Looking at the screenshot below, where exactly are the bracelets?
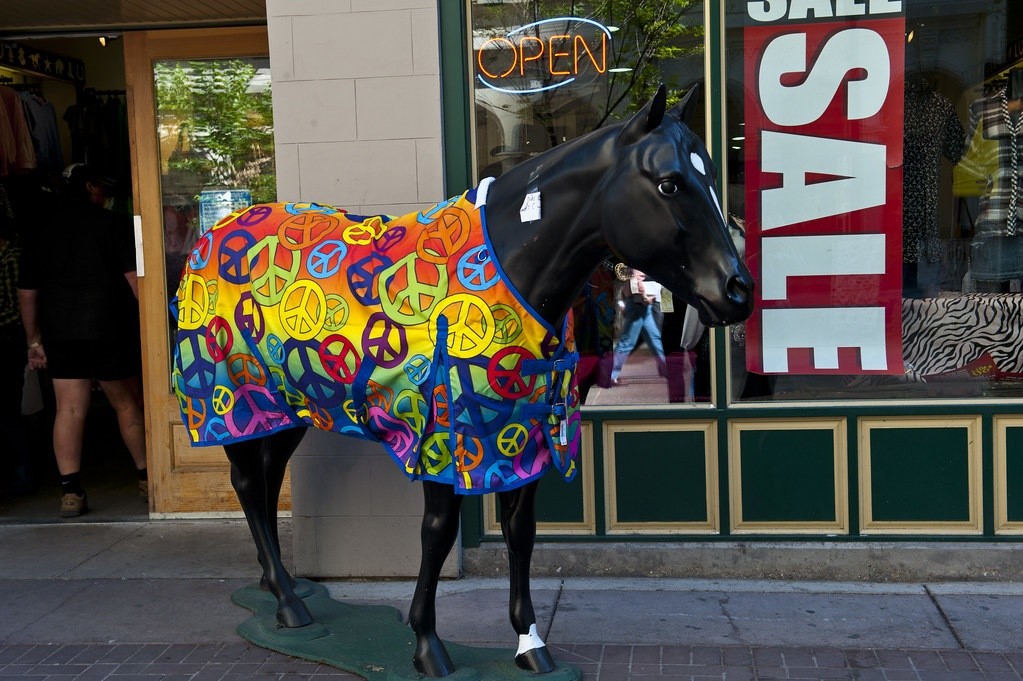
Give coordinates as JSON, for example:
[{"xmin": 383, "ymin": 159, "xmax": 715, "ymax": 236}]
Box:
[{"xmin": 28, "ymin": 340, "xmax": 42, "ymax": 348}]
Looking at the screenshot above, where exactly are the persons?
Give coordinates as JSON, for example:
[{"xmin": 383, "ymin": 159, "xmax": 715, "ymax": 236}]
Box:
[
  {"xmin": 966, "ymin": 79, "xmax": 1023, "ymax": 283},
  {"xmin": 611, "ymin": 269, "xmax": 667, "ymax": 386},
  {"xmin": 18, "ymin": 167, "xmax": 149, "ymax": 518},
  {"xmin": 902, "ymin": 73, "xmax": 967, "ymax": 263}
]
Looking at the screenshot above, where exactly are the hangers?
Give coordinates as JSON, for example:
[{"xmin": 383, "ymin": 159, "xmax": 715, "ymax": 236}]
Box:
[
  {"xmin": 0, "ymin": 74, "xmax": 47, "ymax": 104},
  {"xmin": 92, "ymin": 88, "xmax": 125, "ymax": 103}
]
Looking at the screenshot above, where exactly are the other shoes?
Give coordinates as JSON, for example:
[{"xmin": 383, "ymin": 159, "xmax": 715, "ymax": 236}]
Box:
[
  {"xmin": 60, "ymin": 494, "xmax": 88, "ymax": 516},
  {"xmin": 138, "ymin": 481, "xmax": 149, "ymax": 504},
  {"xmin": 611, "ymin": 377, "xmax": 626, "ymax": 385}
]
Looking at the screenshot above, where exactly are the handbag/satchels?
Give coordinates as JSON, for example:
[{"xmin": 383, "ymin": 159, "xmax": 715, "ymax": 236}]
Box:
[{"xmin": 633, "ymin": 281, "xmax": 662, "ymax": 303}]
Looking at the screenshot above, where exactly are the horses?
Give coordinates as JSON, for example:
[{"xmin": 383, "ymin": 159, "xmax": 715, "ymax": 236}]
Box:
[{"xmin": 171, "ymin": 80, "xmax": 755, "ymax": 679}]
